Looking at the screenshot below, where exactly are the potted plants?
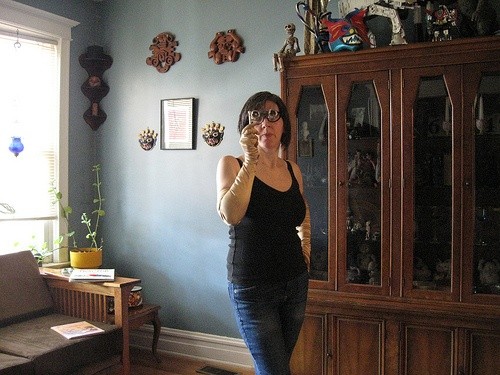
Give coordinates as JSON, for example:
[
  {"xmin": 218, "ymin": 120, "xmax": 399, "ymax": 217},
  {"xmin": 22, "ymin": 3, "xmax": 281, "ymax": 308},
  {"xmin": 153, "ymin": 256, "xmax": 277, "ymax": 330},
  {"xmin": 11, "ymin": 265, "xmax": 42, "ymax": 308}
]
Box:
[{"xmin": 14, "ymin": 164, "xmax": 105, "ymax": 269}]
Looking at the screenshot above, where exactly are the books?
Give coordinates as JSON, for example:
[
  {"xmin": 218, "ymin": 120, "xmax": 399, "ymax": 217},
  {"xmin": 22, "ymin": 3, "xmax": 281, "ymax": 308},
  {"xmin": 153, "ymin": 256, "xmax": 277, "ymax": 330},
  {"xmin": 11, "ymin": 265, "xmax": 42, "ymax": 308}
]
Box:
[
  {"xmin": 51, "ymin": 321, "xmax": 105, "ymax": 339},
  {"xmin": 69, "ymin": 269, "xmax": 115, "ymax": 282}
]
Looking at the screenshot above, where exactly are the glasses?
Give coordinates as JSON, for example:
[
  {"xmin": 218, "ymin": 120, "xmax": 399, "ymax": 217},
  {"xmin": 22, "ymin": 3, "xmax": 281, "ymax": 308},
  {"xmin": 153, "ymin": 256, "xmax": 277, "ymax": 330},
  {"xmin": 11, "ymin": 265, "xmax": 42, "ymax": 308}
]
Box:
[{"xmin": 250, "ymin": 111, "xmax": 282, "ymax": 125}]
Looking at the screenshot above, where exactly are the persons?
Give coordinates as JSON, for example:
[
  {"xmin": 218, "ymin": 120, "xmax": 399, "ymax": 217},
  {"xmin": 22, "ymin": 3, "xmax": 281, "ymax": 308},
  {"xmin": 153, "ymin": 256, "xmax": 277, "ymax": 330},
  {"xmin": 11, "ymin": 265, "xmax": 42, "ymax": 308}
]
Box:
[{"xmin": 217, "ymin": 90, "xmax": 311, "ymax": 374}]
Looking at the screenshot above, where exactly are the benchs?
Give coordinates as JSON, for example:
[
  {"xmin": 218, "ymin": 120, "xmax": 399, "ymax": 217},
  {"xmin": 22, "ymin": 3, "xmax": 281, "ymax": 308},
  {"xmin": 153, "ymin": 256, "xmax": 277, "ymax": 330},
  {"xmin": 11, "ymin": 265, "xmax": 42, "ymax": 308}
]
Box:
[{"xmin": 0, "ymin": 250, "xmax": 141, "ymax": 375}]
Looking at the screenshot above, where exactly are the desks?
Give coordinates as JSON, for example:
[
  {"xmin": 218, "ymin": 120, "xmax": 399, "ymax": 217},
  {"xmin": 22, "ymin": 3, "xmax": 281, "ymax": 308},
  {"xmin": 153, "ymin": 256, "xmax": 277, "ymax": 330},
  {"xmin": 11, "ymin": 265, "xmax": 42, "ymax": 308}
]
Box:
[{"xmin": 107, "ymin": 303, "xmax": 162, "ymax": 364}]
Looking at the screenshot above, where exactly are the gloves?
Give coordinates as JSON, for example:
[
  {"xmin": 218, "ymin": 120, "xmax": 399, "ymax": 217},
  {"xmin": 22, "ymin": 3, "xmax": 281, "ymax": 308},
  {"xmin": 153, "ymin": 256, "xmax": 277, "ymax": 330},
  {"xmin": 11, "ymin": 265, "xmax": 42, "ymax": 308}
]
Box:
[
  {"xmin": 218, "ymin": 121, "xmax": 260, "ymax": 226},
  {"xmin": 296, "ymin": 206, "xmax": 311, "ymax": 272}
]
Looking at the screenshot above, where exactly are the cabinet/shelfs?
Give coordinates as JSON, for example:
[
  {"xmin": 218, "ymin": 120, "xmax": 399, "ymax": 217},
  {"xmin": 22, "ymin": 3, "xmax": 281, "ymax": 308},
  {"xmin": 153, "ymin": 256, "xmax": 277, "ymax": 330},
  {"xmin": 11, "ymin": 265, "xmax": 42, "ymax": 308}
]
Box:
[
  {"xmin": 280, "ymin": 37, "xmax": 500, "ymax": 375},
  {"xmin": 79, "ymin": 44, "xmax": 112, "ymax": 131}
]
